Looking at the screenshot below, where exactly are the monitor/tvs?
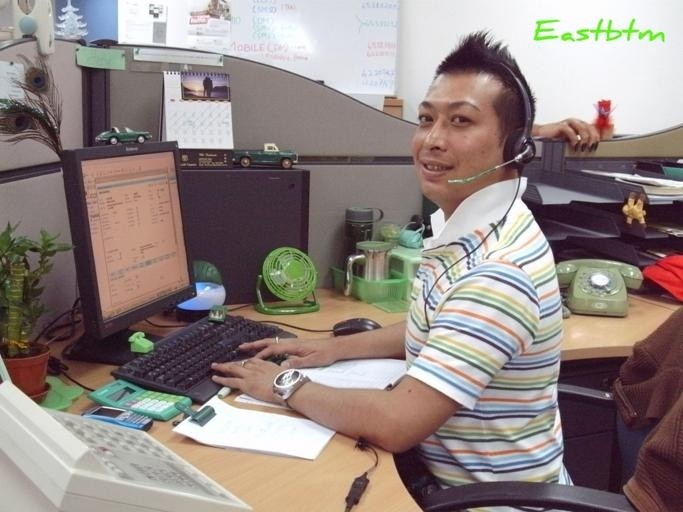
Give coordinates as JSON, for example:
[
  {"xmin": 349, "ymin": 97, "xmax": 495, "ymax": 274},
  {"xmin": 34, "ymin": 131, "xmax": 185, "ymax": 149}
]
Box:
[{"xmin": 58, "ymin": 140, "xmax": 199, "ymax": 366}]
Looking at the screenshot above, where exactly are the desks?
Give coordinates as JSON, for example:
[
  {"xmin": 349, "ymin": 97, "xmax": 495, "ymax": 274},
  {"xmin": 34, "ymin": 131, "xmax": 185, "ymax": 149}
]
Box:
[{"xmin": 32, "ymin": 270, "xmax": 683, "ymax": 512}]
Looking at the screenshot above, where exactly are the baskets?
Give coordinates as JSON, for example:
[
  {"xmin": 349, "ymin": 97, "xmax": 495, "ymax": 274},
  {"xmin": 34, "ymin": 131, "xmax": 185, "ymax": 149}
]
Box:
[{"xmin": 329, "ymin": 266, "xmax": 409, "ymax": 303}]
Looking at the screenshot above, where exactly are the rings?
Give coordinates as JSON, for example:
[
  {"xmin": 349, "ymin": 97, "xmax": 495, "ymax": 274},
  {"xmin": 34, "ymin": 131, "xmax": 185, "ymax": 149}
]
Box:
[
  {"xmin": 276, "ymin": 336, "xmax": 279, "ymax": 344},
  {"xmin": 242, "ymin": 360, "xmax": 250, "ymax": 366}
]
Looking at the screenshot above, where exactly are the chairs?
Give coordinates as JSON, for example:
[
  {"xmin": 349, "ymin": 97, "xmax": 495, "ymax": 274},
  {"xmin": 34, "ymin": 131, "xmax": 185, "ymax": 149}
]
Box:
[{"xmin": 418, "ymin": 304, "xmax": 683, "ymax": 511}]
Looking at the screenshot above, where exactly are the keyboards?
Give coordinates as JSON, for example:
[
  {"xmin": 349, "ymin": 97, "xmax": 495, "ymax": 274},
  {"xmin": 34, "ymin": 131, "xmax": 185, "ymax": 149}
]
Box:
[{"xmin": 109, "ymin": 312, "xmax": 297, "ymax": 405}]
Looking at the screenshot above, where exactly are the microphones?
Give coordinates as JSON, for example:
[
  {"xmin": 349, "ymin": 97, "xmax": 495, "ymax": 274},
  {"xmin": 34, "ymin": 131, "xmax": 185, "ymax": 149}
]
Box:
[{"xmin": 447, "ymin": 159, "xmax": 513, "ymax": 186}]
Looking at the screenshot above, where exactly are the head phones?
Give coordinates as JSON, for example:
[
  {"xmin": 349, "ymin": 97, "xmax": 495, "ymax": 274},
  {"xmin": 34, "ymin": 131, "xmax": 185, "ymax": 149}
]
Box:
[{"xmin": 500, "ymin": 61, "xmax": 539, "ymax": 173}]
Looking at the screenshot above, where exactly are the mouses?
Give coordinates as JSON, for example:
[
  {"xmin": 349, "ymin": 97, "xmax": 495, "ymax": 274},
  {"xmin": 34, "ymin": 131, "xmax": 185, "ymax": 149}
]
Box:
[{"xmin": 333, "ymin": 315, "xmax": 380, "ymax": 336}]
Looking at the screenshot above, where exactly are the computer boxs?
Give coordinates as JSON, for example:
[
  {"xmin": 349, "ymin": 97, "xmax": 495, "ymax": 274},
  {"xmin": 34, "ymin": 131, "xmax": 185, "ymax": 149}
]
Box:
[{"xmin": 173, "ymin": 166, "xmax": 310, "ymax": 302}]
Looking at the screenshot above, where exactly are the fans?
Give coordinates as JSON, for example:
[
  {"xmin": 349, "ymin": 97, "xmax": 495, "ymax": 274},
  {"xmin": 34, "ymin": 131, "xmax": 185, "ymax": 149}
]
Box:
[{"xmin": 255, "ymin": 248, "xmax": 321, "ymax": 313}]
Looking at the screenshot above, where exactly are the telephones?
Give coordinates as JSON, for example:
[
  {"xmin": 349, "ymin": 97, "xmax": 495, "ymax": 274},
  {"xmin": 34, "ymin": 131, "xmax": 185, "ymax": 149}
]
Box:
[
  {"xmin": 556, "ymin": 257, "xmax": 643, "ymax": 318},
  {"xmin": 128, "ymin": 331, "xmax": 154, "ymax": 353}
]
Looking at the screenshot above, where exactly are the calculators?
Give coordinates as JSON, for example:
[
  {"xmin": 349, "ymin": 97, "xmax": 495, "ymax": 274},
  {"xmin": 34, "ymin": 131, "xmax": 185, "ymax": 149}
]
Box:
[{"xmin": 89, "ymin": 380, "xmax": 191, "ymax": 420}]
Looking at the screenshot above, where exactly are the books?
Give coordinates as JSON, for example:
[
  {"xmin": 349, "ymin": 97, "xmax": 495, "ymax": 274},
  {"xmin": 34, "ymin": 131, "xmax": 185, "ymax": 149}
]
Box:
[{"xmin": 585, "ymin": 170, "xmax": 683, "ymax": 199}]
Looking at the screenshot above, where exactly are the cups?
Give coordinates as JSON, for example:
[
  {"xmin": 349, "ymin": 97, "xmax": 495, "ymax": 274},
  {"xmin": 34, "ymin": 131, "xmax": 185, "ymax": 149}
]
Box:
[{"xmin": 343, "ymin": 240, "xmax": 394, "ymax": 297}]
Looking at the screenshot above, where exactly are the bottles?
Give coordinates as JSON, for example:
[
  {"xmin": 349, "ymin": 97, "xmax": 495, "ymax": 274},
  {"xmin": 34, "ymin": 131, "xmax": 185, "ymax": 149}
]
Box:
[{"xmin": 342, "ymin": 206, "xmax": 384, "ymax": 276}]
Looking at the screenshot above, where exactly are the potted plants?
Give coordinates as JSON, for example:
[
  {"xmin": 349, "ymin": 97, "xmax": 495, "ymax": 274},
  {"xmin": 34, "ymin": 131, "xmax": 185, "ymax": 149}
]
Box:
[{"xmin": 0, "ymin": 221, "xmax": 76, "ymax": 404}]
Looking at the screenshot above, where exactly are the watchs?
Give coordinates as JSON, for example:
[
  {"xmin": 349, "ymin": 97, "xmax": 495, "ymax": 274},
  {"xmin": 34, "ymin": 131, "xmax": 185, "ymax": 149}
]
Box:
[{"xmin": 272, "ymin": 369, "xmax": 312, "ymax": 409}]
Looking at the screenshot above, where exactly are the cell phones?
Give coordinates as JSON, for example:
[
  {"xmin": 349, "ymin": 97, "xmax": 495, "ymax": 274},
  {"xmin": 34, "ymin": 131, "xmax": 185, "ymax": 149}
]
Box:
[{"xmin": 81, "ymin": 404, "xmax": 153, "ymax": 430}]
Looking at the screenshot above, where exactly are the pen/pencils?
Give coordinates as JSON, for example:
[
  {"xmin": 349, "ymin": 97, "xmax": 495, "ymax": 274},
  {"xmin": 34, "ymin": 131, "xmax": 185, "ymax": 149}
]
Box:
[{"xmin": 218, "ymin": 386, "xmax": 232, "ymax": 399}]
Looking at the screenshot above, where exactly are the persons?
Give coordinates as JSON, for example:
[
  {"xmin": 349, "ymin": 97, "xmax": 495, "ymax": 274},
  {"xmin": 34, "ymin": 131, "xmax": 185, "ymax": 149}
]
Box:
[
  {"xmin": 531, "ymin": 119, "xmax": 600, "ymax": 153},
  {"xmin": 211, "ymin": 23, "xmax": 575, "ymax": 512},
  {"xmin": 94, "ymin": 127, "xmax": 152, "ymax": 145},
  {"xmin": 232, "ymin": 143, "xmax": 296, "ymax": 165},
  {"xmin": 203, "ymin": 76, "xmax": 213, "ymax": 98}
]
